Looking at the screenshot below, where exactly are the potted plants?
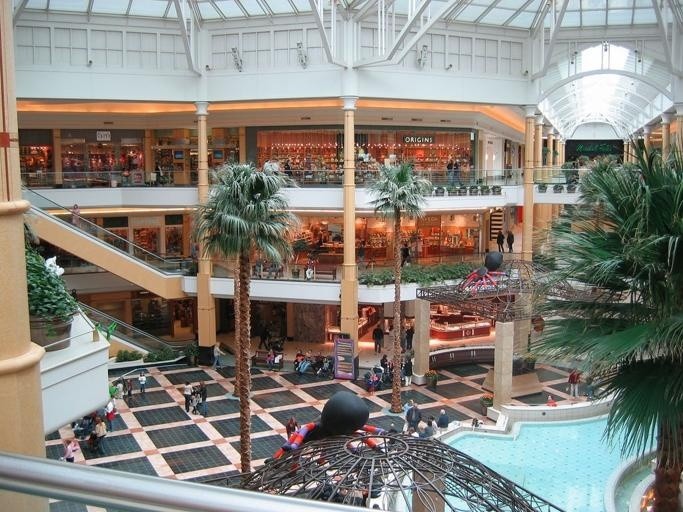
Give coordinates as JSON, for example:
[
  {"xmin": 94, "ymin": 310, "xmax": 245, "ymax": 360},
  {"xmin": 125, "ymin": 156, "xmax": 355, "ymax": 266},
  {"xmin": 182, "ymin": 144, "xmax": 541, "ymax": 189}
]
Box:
[
  {"xmin": 291, "ymin": 239, "xmax": 308, "ymax": 278},
  {"xmin": 538, "ymin": 183, "xmax": 577, "ymax": 193},
  {"xmin": 436, "ymin": 185, "xmax": 501, "ymax": 196},
  {"xmin": 26, "ymin": 244, "xmax": 79, "ymax": 352},
  {"xmin": 186, "ymin": 341, "xmax": 199, "ymax": 363}
]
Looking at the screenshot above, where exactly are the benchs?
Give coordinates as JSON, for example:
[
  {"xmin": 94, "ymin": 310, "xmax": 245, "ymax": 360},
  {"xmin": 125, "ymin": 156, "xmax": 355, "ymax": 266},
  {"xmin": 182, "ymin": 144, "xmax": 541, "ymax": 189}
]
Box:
[
  {"xmin": 293, "ymin": 356, "xmax": 335, "ymax": 379},
  {"xmin": 302, "ymin": 263, "xmax": 337, "ymax": 280},
  {"xmin": 252, "ymin": 261, "xmax": 284, "ymax": 279},
  {"xmin": 251, "ymin": 351, "xmax": 284, "ymax": 370}
]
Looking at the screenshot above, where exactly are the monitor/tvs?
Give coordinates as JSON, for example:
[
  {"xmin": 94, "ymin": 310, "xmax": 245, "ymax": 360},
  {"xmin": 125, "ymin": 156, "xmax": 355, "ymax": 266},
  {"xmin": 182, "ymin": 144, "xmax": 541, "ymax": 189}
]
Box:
[
  {"xmin": 174, "ymin": 151, "xmax": 184, "ymax": 159},
  {"xmin": 213, "ymin": 150, "xmax": 223, "ymax": 159}
]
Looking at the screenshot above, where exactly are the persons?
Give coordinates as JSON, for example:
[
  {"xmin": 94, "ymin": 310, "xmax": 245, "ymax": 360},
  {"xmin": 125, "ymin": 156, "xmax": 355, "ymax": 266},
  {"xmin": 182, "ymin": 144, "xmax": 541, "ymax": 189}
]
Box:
[
  {"xmin": 497, "ymin": 230, "xmax": 515, "ymax": 253},
  {"xmin": 287, "ymin": 418, "xmax": 301, "ymax": 439},
  {"xmin": 72, "ymin": 204, "xmax": 81, "ymax": 227},
  {"xmin": 547, "ymin": 395, "xmax": 556, "ymax": 406},
  {"xmin": 184, "ymin": 380, "xmax": 208, "ymax": 416},
  {"xmin": 262, "ymin": 157, "xmax": 292, "ymax": 178},
  {"xmin": 446, "ymin": 160, "xmax": 464, "ymax": 187},
  {"xmin": 568, "ymin": 369, "xmax": 593, "ymax": 398},
  {"xmin": 258, "ymin": 321, "xmax": 334, "ymax": 377},
  {"xmin": 358, "ymin": 244, "xmax": 364, "ymax": 265},
  {"xmin": 365, "ymin": 322, "xmax": 416, "ymax": 391},
  {"xmin": 155, "ymin": 162, "xmax": 164, "ymax": 185},
  {"xmin": 211, "ymin": 342, "xmax": 227, "ymax": 370},
  {"xmin": 401, "ymin": 244, "xmax": 412, "ymax": 267},
  {"xmin": 70, "ymin": 289, "xmax": 78, "ymax": 302},
  {"xmin": 390, "ymin": 398, "xmax": 448, "ymax": 438},
  {"xmin": 63, "ymin": 369, "xmax": 148, "ymax": 462}
]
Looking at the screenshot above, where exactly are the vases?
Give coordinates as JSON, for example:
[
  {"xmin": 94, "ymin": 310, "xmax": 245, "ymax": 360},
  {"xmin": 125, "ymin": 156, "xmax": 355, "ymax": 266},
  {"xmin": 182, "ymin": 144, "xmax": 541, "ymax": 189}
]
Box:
[
  {"xmin": 527, "ymin": 361, "xmax": 534, "ymax": 369},
  {"xmin": 426, "ymin": 376, "xmax": 437, "ymax": 388},
  {"xmin": 481, "ymin": 406, "xmax": 487, "ymax": 416}
]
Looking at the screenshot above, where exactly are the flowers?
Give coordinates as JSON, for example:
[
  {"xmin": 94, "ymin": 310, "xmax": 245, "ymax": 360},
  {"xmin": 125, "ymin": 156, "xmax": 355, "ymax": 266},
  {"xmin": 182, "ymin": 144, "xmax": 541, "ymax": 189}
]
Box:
[
  {"xmin": 425, "ymin": 370, "xmax": 437, "ymax": 377},
  {"xmin": 479, "ymin": 394, "xmax": 494, "ymax": 407},
  {"xmin": 525, "ymin": 353, "xmax": 537, "ymax": 362}
]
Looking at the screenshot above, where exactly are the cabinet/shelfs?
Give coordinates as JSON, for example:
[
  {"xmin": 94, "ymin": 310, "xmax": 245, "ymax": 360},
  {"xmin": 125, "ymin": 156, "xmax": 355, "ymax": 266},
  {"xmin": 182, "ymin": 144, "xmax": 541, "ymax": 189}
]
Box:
[{"xmin": 258, "ymin": 146, "xmax": 469, "ymax": 178}]
